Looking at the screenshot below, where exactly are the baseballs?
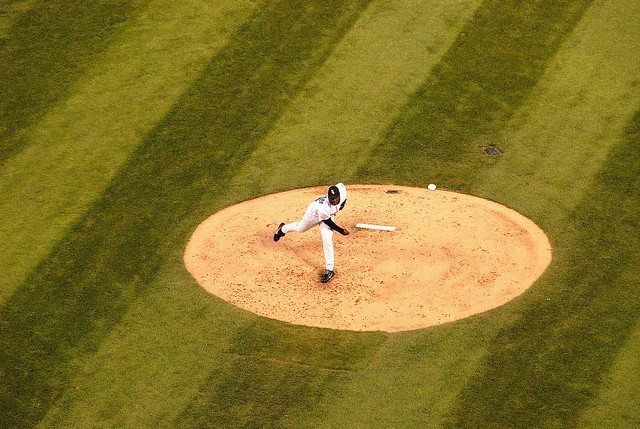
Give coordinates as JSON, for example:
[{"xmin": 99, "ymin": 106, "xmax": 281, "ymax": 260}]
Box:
[{"xmin": 428, "ymin": 184, "xmax": 436, "ymax": 190}]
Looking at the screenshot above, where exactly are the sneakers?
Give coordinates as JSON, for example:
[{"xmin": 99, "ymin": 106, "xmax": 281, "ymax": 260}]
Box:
[
  {"xmin": 321, "ymin": 269, "xmax": 333, "ymax": 282},
  {"xmin": 274, "ymin": 223, "xmax": 285, "ymax": 241}
]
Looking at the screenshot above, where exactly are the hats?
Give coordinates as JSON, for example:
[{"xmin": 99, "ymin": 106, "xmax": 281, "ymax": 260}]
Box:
[{"xmin": 328, "ymin": 186, "xmax": 339, "ymax": 198}]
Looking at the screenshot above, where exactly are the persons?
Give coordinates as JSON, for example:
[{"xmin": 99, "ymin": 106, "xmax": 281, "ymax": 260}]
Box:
[{"xmin": 273, "ymin": 183, "xmax": 349, "ymax": 283}]
